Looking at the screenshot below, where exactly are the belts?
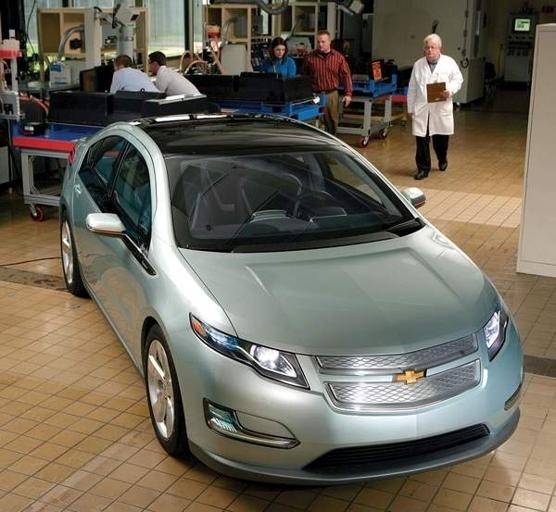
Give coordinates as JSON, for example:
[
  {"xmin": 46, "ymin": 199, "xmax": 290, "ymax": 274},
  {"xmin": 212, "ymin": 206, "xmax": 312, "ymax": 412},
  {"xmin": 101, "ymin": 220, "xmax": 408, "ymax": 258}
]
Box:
[{"xmin": 313, "ymin": 88, "xmax": 337, "ymax": 94}]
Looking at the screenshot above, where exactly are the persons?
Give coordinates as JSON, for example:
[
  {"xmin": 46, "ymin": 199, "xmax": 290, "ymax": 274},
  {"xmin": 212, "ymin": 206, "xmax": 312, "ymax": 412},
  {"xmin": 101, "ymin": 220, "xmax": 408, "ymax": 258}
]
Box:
[
  {"xmin": 262, "ymin": 38, "xmax": 296, "ymax": 118},
  {"xmin": 110, "ymin": 54, "xmax": 157, "ymax": 94},
  {"xmin": 300, "ymin": 30, "xmax": 354, "ymax": 136},
  {"xmin": 148, "ymin": 51, "xmax": 200, "ymax": 96},
  {"xmin": 406, "ymin": 32, "xmax": 464, "ymax": 180}
]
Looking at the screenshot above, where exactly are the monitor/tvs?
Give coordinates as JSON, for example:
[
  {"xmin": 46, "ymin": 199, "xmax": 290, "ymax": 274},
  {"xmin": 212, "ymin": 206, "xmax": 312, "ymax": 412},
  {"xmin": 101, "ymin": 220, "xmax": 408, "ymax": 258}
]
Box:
[
  {"xmin": 80, "ymin": 69, "xmax": 98, "ymax": 92},
  {"xmin": 508, "ymin": 13, "xmax": 536, "ymax": 43}
]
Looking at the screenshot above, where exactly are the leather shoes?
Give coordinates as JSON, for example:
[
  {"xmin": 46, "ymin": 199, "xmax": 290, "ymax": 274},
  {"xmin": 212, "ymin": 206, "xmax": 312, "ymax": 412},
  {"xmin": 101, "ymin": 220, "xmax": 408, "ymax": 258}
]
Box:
[
  {"xmin": 414, "ymin": 168, "xmax": 430, "ymax": 180},
  {"xmin": 437, "ymin": 157, "xmax": 449, "ymax": 171}
]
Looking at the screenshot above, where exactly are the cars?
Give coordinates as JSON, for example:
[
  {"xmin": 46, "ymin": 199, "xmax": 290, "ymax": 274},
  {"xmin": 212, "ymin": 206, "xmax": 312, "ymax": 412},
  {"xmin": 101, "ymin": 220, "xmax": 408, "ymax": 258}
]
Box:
[{"xmin": 57, "ymin": 111, "xmax": 526, "ymax": 483}]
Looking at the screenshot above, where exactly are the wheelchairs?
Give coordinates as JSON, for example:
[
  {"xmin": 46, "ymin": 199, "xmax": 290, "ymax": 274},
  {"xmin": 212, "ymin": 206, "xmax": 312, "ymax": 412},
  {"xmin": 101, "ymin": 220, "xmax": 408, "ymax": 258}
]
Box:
[{"xmin": 481, "ymin": 57, "xmax": 498, "ymax": 101}]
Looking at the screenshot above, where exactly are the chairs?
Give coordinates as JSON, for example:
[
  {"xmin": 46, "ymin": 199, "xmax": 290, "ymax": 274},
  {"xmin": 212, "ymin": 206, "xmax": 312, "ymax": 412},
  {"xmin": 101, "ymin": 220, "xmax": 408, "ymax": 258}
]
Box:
[{"xmin": 179, "ymin": 156, "xmax": 304, "ymax": 225}]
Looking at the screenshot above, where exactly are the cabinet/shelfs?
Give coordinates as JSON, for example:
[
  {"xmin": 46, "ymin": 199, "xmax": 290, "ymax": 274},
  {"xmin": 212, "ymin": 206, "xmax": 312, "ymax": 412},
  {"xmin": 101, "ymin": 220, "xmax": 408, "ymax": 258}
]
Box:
[
  {"xmin": 271, "ymin": 1, "xmax": 335, "ymax": 51},
  {"xmin": 35, "ymin": 6, "xmax": 149, "ymax": 80},
  {"xmin": 199, "ymin": 2, "xmax": 278, "ymax": 72}
]
